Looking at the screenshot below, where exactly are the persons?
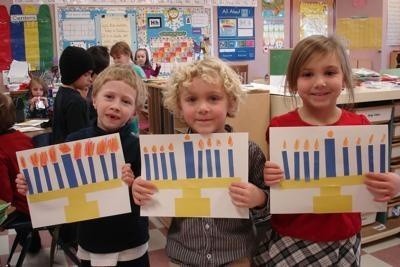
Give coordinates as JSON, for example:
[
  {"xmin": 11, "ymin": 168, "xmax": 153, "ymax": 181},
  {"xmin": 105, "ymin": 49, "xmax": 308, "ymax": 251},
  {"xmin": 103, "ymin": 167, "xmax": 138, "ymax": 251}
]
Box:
[
  {"xmin": 23, "ymin": 77, "xmax": 55, "ymax": 119},
  {"xmin": 109, "ymin": 41, "xmax": 146, "ymax": 135},
  {"xmin": 51, "ymin": 45, "xmax": 94, "ymax": 141},
  {"xmin": 16, "ymin": 63, "xmax": 151, "ymax": 266},
  {"xmin": 134, "ymin": 47, "xmax": 161, "ymax": 131},
  {"xmin": 253, "ymin": 34, "xmax": 400, "ymax": 266},
  {"xmin": 0, "ymin": 91, "xmax": 42, "ymax": 255},
  {"xmin": 86, "ymin": 45, "xmax": 110, "ymax": 100},
  {"xmin": 132, "ymin": 54, "xmax": 270, "ymax": 266}
]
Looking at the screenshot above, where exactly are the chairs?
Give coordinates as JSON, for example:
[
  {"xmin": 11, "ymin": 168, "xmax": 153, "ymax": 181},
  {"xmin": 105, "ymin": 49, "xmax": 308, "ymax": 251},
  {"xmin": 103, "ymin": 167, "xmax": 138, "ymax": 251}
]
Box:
[{"xmin": 0, "ymin": 129, "xmax": 84, "ymax": 267}]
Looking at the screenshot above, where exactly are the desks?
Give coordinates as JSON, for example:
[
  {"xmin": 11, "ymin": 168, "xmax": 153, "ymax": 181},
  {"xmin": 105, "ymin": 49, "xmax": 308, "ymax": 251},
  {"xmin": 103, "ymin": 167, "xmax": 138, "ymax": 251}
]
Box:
[{"xmin": 12, "ymin": 119, "xmax": 54, "ymax": 140}]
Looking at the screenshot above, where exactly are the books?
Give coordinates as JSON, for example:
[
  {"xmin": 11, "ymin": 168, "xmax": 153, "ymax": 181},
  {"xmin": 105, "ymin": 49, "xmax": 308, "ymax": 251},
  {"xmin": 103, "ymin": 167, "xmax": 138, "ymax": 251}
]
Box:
[{"xmin": 15, "ymin": 118, "xmax": 50, "ymax": 126}]
